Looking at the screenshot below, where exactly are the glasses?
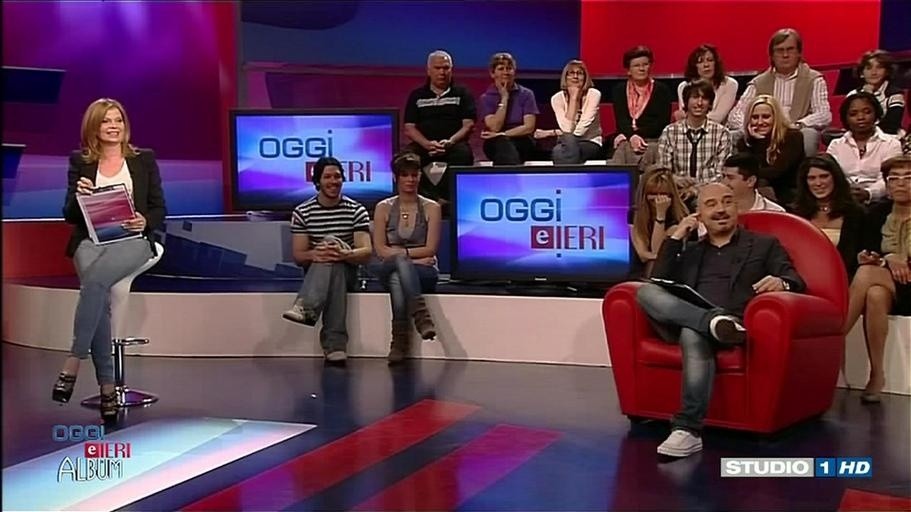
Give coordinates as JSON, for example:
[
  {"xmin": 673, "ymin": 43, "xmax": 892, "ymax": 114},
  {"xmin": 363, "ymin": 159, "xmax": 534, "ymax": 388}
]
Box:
[{"xmin": 886, "ymin": 175, "xmax": 911, "ymax": 183}]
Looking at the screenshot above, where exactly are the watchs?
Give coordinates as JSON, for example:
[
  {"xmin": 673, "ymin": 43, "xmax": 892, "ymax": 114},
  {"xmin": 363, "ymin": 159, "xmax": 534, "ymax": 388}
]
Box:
[
  {"xmin": 781, "ymin": 279, "xmax": 791, "ymax": 290},
  {"xmin": 655, "ymin": 219, "xmax": 666, "ymax": 225}
]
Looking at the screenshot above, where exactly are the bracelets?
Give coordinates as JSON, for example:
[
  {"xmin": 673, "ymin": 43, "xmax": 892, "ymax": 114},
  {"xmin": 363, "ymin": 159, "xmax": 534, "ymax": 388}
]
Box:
[
  {"xmin": 554, "ymin": 129, "xmax": 558, "ymax": 136},
  {"xmin": 498, "ymin": 104, "xmax": 507, "ymax": 107}
]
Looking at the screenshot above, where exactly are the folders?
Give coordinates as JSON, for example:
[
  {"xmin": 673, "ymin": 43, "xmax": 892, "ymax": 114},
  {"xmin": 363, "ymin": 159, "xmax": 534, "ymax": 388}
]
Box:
[{"xmin": 75, "ymin": 182, "xmax": 143, "ymax": 246}]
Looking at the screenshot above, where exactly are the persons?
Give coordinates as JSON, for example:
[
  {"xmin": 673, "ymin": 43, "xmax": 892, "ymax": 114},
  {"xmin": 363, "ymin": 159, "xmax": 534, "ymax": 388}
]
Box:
[
  {"xmin": 480, "ymin": 53, "xmax": 537, "ymax": 165},
  {"xmin": 825, "ymin": 92, "xmax": 903, "ymax": 205},
  {"xmin": 658, "ymin": 80, "xmax": 731, "ymax": 201},
  {"xmin": 841, "ymin": 155, "xmax": 911, "ymax": 405},
  {"xmin": 51, "ymin": 98, "xmax": 167, "ymax": 422},
  {"xmin": 607, "ymin": 45, "xmax": 672, "ymax": 165},
  {"xmin": 533, "ymin": 60, "xmax": 603, "ymax": 167},
  {"xmin": 733, "ymin": 94, "xmax": 806, "ymax": 201},
  {"xmin": 393, "ymin": 49, "xmax": 477, "ymax": 218},
  {"xmin": 280, "ymin": 156, "xmax": 373, "ymax": 363},
  {"xmin": 726, "ymin": 28, "xmax": 832, "ymax": 129},
  {"xmin": 628, "ymin": 169, "xmax": 699, "ymax": 267},
  {"xmin": 786, "ymin": 153, "xmax": 883, "ymax": 283},
  {"xmin": 637, "ymin": 182, "xmax": 807, "ymax": 457},
  {"xmin": 844, "ymin": 49, "xmax": 905, "ymax": 135},
  {"xmin": 374, "ymin": 150, "xmax": 441, "ymax": 363},
  {"xmin": 674, "ymin": 46, "xmax": 740, "ymax": 126},
  {"xmin": 718, "ymin": 157, "xmax": 783, "ymax": 211}
]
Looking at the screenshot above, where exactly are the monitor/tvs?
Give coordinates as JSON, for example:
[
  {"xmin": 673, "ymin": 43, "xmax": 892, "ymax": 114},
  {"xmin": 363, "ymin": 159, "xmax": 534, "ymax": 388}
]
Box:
[
  {"xmin": 446, "ymin": 164, "xmax": 637, "ymax": 293},
  {"xmin": 226, "ymin": 107, "xmax": 398, "ymax": 213}
]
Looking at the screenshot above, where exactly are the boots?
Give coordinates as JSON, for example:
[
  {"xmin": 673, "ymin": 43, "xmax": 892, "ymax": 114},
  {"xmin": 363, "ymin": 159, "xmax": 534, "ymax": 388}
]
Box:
[
  {"xmin": 409, "ymin": 295, "xmax": 436, "ymax": 341},
  {"xmin": 387, "ymin": 321, "xmax": 410, "ymax": 362}
]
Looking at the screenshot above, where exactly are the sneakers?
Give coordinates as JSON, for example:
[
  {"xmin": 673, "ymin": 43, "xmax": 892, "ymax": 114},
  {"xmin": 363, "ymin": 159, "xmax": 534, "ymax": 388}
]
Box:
[
  {"xmin": 657, "ymin": 430, "xmax": 702, "ymax": 457},
  {"xmin": 710, "ymin": 316, "xmax": 745, "ymax": 345},
  {"xmin": 282, "ymin": 299, "xmax": 315, "ymax": 327},
  {"xmin": 326, "ymin": 351, "xmax": 347, "ymax": 362}
]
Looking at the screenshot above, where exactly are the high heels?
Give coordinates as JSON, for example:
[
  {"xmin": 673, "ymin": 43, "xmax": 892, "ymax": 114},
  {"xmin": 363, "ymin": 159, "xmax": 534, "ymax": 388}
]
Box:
[
  {"xmin": 861, "ymin": 376, "xmax": 886, "ymax": 403},
  {"xmin": 101, "ymin": 387, "xmax": 120, "ymax": 421},
  {"xmin": 51, "ymin": 372, "xmax": 78, "ymax": 404}
]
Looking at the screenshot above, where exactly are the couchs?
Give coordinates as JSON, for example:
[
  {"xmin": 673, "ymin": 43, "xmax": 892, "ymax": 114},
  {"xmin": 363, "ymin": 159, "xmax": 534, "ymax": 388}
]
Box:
[{"xmin": 601, "ymin": 209, "xmax": 849, "ymax": 438}]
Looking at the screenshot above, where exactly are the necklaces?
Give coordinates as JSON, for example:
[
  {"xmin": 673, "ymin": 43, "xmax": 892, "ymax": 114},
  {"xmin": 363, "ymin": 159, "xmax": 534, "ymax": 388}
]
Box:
[
  {"xmin": 399, "ymin": 202, "xmax": 417, "ymax": 220},
  {"xmin": 817, "ymin": 206, "xmax": 830, "ymax": 212}
]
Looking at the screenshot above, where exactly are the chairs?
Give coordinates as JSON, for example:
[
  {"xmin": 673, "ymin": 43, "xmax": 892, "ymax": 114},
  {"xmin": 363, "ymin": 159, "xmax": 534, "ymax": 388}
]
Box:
[{"xmin": 79, "ymin": 241, "xmax": 163, "ymax": 407}]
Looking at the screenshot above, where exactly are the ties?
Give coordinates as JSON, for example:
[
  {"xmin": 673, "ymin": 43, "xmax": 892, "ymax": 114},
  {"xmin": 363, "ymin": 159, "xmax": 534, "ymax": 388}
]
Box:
[{"xmin": 687, "ymin": 130, "xmax": 705, "ymax": 178}]
